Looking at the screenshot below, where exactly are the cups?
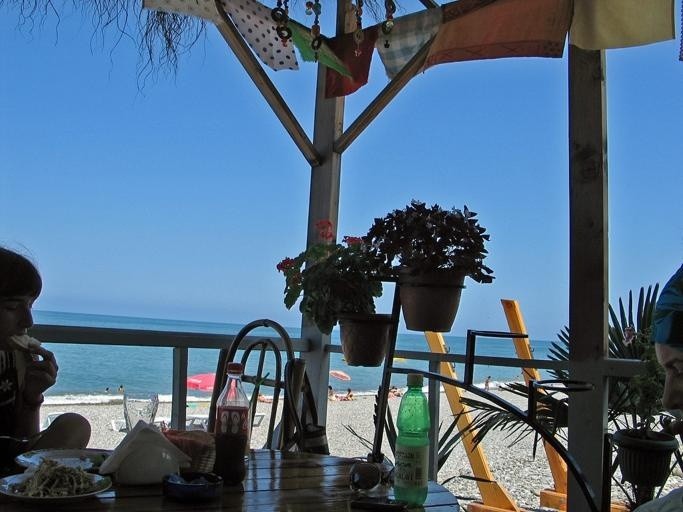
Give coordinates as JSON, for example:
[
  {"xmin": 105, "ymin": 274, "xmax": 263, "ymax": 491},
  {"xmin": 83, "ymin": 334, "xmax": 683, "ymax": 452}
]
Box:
[{"xmin": 123, "ymin": 392, "xmax": 158, "ymax": 430}]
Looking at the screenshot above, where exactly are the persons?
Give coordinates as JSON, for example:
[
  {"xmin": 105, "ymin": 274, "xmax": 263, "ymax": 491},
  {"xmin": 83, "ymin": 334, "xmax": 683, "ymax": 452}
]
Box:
[
  {"xmin": 631, "ymin": 264, "xmax": 683, "ymax": 512},
  {"xmin": 327, "ymin": 386, "xmax": 402, "ymax": 401},
  {"xmin": 118, "ymin": 385, "xmax": 124, "ymax": 393},
  {"xmin": 258, "ymin": 395, "xmax": 273, "ymax": 403},
  {"xmin": 485, "ymin": 376, "xmax": 491, "ymax": 391},
  {"xmin": 0, "ymin": 245, "xmax": 91, "ymax": 460},
  {"xmin": 105, "ymin": 388, "xmax": 111, "ymax": 393}
]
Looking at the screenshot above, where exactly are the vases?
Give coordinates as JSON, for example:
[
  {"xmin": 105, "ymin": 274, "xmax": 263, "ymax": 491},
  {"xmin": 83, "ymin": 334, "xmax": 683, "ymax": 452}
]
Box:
[{"xmin": 331, "ymin": 307, "xmax": 393, "ymax": 367}]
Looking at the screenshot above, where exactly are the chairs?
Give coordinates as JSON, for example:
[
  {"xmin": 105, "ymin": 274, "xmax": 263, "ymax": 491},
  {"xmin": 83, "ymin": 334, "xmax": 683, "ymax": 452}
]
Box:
[{"xmin": 205, "ymin": 318, "xmax": 307, "ymax": 450}]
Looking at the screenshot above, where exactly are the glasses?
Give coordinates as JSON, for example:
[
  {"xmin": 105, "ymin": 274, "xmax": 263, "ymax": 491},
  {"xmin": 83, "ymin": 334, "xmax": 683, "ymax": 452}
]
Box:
[{"xmin": 348, "ymin": 453, "xmax": 385, "ymax": 498}]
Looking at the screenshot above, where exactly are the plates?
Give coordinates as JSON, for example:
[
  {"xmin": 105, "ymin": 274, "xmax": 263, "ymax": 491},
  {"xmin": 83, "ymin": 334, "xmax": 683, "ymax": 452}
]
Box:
[{"xmin": 0, "ymin": 447, "xmax": 112, "ymax": 502}]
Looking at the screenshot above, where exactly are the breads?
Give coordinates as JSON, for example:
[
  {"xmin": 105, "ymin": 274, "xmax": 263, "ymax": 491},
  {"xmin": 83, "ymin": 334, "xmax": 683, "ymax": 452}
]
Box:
[{"xmin": 7, "ymin": 334, "xmax": 42, "ymax": 350}]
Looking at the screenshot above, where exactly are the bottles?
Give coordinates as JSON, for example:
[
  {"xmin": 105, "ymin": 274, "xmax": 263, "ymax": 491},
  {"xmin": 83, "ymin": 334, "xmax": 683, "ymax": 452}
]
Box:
[
  {"xmin": 395, "ymin": 375, "xmax": 431, "ymax": 508},
  {"xmin": 215, "ymin": 362, "xmax": 250, "ymax": 486},
  {"xmin": 348, "ymin": 453, "xmax": 391, "ymax": 497}
]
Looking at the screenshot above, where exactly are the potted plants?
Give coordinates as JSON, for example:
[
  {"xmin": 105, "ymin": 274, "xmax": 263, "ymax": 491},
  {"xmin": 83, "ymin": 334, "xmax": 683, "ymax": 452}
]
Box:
[
  {"xmin": 442, "ymin": 280, "xmax": 682, "ymax": 488},
  {"xmin": 362, "ymin": 198, "xmax": 496, "ymax": 333}
]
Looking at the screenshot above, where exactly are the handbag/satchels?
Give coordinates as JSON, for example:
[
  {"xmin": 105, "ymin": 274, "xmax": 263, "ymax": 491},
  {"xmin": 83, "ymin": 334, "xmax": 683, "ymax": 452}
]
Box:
[{"xmin": 279, "ymin": 426, "xmax": 330, "ymax": 456}]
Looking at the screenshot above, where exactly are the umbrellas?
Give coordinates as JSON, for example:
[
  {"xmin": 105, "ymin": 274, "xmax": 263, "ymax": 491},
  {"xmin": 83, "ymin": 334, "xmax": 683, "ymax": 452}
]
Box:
[
  {"xmin": 330, "ymin": 371, "xmax": 350, "ymax": 381},
  {"xmin": 185, "ymin": 372, "xmax": 237, "ymax": 391}
]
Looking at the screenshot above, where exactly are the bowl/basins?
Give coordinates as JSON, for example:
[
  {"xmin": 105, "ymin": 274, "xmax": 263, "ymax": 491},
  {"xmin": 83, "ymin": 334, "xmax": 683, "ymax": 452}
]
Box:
[{"xmin": 161, "ymin": 470, "xmax": 222, "ymax": 501}]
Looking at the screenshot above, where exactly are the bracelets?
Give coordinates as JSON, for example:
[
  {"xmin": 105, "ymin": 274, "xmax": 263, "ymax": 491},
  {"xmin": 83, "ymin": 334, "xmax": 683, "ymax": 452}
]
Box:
[{"xmin": 21, "ymin": 390, "xmax": 44, "ymax": 407}]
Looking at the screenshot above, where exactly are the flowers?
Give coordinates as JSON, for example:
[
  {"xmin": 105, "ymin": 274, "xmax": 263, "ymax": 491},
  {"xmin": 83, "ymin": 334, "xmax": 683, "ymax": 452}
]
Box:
[{"xmin": 268, "ymin": 216, "xmax": 382, "ymax": 337}]
